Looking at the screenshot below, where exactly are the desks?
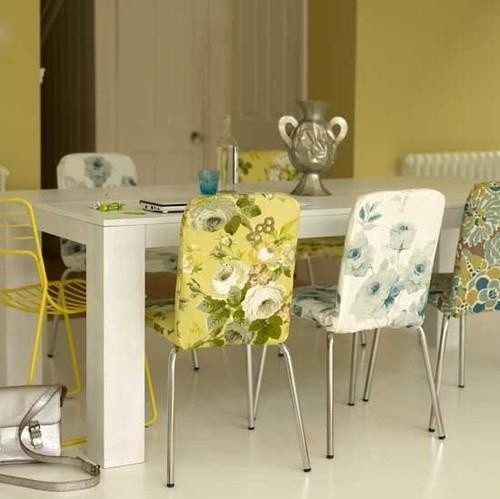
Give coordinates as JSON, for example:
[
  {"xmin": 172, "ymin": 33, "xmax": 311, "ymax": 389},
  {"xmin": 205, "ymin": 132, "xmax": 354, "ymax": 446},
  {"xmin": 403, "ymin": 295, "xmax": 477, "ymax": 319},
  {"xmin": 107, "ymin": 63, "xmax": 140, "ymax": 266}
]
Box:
[{"xmin": 0, "ymin": 185, "xmax": 500, "ymax": 470}]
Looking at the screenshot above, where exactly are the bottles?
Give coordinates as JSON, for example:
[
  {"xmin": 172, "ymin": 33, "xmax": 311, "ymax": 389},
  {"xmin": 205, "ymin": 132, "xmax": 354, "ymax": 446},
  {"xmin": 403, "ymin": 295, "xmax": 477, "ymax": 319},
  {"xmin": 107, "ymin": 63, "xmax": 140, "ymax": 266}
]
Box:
[{"xmin": 217, "ymin": 113, "xmax": 239, "ymax": 192}]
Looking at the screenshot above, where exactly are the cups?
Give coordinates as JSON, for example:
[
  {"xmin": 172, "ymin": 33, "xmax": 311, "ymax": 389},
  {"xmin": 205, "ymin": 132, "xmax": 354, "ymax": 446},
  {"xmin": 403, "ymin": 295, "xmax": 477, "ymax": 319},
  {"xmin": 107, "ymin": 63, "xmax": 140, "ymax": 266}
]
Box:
[{"xmin": 199, "ymin": 170, "xmax": 220, "ymax": 195}]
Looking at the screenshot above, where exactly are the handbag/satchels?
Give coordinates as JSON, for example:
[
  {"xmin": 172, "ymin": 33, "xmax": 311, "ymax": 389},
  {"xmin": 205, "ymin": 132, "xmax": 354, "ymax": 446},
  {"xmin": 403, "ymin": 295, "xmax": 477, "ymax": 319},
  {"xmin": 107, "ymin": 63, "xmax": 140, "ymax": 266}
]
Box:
[{"xmin": 0, "ymin": 383, "xmax": 67, "ymax": 464}]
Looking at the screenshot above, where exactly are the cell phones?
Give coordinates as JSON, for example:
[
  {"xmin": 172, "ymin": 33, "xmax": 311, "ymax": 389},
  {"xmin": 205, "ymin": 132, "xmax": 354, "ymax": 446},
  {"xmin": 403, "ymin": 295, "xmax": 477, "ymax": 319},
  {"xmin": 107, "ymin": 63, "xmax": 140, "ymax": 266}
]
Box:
[{"xmin": 139, "ymin": 199, "xmax": 187, "ymax": 214}]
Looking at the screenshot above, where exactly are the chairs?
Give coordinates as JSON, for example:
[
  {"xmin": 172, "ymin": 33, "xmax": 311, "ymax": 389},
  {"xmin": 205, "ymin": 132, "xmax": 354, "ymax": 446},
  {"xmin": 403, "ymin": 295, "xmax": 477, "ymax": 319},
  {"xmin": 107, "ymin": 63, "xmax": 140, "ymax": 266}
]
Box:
[
  {"xmin": 363, "ymin": 180, "xmax": 500, "ymax": 437},
  {"xmin": 135, "ymin": 191, "xmax": 313, "ymax": 488},
  {"xmin": 219, "ymin": 151, "xmax": 349, "ymax": 282},
  {"xmin": 48, "ymin": 151, "xmax": 201, "ymax": 373},
  {"xmin": 242, "ymin": 191, "xmax": 449, "ymax": 456},
  {"xmin": 1, "ymin": 197, "xmax": 160, "ymax": 451}
]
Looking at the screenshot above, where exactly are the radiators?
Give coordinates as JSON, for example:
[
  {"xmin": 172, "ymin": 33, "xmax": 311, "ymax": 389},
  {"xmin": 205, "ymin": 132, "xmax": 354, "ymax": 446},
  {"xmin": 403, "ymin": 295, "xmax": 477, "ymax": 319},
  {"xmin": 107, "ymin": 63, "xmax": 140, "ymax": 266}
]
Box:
[{"xmin": 403, "ymin": 148, "xmax": 500, "ymax": 175}]
